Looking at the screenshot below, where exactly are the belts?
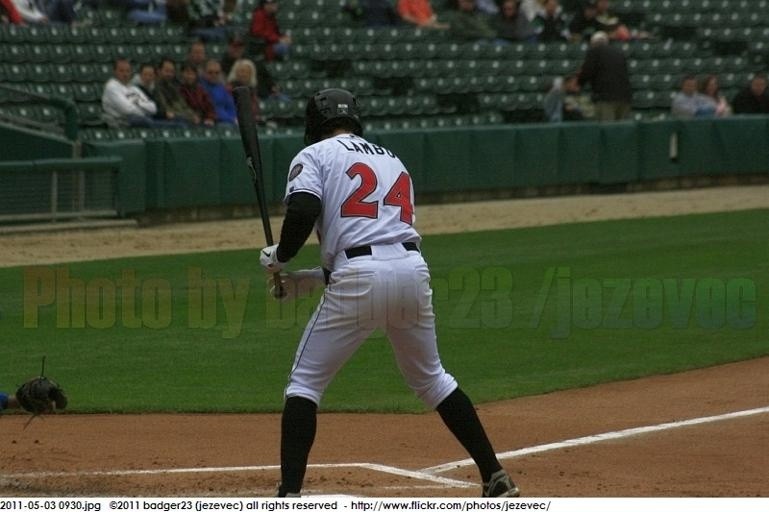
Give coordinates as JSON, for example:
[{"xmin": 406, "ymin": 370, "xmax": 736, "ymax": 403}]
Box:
[{"xmin": 323, "ymin": 242, "xmax": 420, "ymax": 285}]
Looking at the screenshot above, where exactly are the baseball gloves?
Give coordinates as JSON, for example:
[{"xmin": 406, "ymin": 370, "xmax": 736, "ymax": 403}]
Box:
[{"xmin": 15, "ymin": 378, "xmax": 66, "ymax": 415}]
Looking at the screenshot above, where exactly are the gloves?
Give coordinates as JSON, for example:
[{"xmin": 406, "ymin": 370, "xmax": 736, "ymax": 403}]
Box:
[
  {"xmin": 259, "ymin": 243, "xmax": 290, "ymax": 275},
  {"xmin": 265, "ymin": 265, "xmax": 327, "ymax": 303}
]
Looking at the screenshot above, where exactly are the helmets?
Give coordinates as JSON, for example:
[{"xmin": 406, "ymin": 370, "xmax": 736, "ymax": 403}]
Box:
[{"xmin": 304, "ymin": 87, "xmax": 363, "ymax": 146}]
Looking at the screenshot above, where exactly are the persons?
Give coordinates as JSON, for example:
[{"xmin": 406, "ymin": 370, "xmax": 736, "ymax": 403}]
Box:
[
  {"xmin": 1, "ymin": 1, "xmax": 768, "ymax": 130},
  {"xmin": 0, "ymin": 377, "xmax": 68, "ymax": 414},
  {"xmin": 259, "ymin": 87, "xmax": 521, "ymax": 498}
]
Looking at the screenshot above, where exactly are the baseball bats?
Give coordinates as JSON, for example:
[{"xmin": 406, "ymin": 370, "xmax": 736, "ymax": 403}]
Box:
[{"xmin": 232, "ymin": 86, "xmax": 289, "ymax": 299}]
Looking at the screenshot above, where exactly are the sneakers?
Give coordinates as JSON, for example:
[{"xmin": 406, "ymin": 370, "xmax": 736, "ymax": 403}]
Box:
[{"xmin": 482, "ymin": 468, "xmax": 521, "ymax": 498}]
